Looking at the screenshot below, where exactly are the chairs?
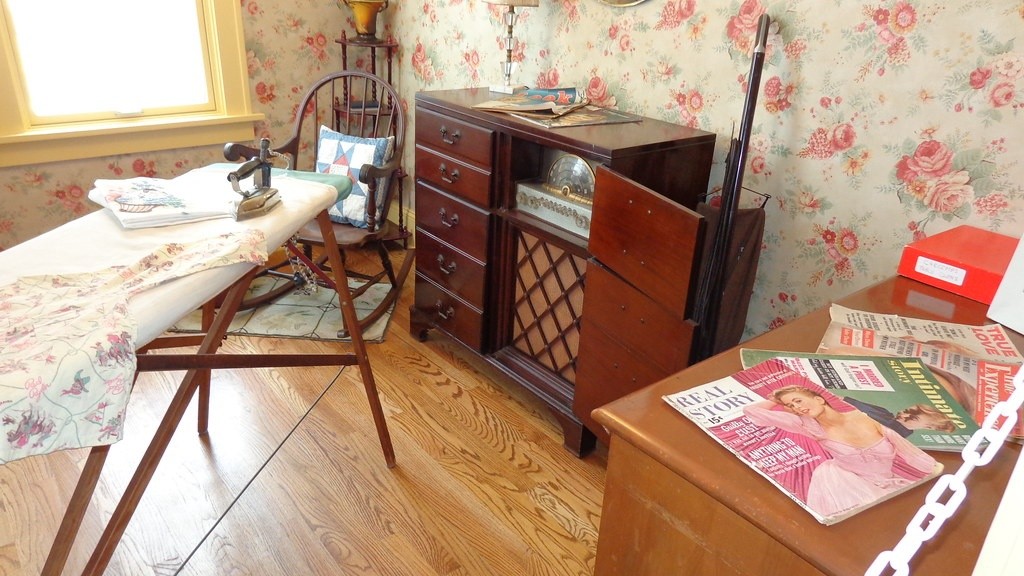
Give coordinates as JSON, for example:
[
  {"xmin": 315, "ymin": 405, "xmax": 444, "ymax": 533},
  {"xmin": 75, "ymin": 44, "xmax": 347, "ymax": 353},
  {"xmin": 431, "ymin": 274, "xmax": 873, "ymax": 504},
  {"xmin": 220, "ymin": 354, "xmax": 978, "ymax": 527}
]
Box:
[{"xmin": 196, "ymin": 70, "xmax": 407, "ymax": 337}]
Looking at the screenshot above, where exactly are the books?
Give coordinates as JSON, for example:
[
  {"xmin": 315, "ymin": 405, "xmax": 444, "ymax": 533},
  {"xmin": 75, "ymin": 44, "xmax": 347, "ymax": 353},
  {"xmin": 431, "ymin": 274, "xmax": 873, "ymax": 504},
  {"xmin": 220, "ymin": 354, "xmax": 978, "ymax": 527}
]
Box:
[
  {"xmin": 663, "ymin": 357, "xmax": 944, "ymax": 525},
  {"xmin": 817, "ymin": 321, "xmax": 1024, "ymax": 447},
  {"xmin": 897, "ymin": 223, "xmax": 1020, "ymax": 305},
  {"xmin": 741, "ymin": 347, "xmax": 990, "ymax": 452},
  {"xmin": 825, "ymin": 304, "xmax": 1024, "ymax": 365},
  {"xmin": 510, "ymin": 104, "xmax": 642, "ymax": 128},
  {"xmin": 472, "ymin": 87, "xmax": 591, "ymax": 120},
  {"xmin": 94, "ymin": 177, "xmax": 233, "ymax": 229}
]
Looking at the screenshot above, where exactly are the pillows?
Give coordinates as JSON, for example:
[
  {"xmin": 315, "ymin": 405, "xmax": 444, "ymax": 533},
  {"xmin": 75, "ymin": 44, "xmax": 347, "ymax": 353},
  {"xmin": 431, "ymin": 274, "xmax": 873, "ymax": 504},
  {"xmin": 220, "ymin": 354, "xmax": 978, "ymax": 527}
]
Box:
[{"xmin": 316, "ymin": 124, "xmax": 395, "ymax": 230}]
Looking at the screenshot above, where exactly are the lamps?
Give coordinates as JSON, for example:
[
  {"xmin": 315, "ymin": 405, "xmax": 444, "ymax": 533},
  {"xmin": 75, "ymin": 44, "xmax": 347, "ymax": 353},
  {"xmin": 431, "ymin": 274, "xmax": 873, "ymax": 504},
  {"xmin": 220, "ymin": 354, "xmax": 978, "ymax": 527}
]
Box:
[{"xmin": 481, "ymin": 0, "xmax": 539, "ymax": 94}]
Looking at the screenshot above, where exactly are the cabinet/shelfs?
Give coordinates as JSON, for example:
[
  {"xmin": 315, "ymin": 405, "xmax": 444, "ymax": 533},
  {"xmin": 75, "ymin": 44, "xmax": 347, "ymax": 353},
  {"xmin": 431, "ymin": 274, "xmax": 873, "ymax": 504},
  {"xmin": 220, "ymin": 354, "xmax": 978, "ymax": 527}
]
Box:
[
  {"xmin": 332, "ymin": 30, "xmax": 413, "ymax": 250},
  {"xmin": 408, "ymin": 86, "xmax": 716, "ymax": 459}
]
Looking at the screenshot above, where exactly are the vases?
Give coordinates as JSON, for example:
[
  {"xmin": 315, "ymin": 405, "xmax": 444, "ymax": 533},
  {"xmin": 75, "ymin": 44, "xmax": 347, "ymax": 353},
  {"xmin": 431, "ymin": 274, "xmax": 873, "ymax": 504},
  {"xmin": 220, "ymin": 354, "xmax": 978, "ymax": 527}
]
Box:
[{"xmin": 341, "ymin": 0, "xmax": 389, "ymax": 44}]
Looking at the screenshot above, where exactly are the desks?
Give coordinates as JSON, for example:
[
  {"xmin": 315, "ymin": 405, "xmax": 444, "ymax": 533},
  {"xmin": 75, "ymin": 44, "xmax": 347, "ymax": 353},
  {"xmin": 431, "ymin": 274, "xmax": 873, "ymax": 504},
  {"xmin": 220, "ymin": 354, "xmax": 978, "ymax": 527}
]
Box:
[
  {"xmin": 0, "ymin": 163, "xmax": 396, "ymax": 576},
  {"xmin": 591, "ymin": 273, "xmax": 1024, "ymax": 576}
]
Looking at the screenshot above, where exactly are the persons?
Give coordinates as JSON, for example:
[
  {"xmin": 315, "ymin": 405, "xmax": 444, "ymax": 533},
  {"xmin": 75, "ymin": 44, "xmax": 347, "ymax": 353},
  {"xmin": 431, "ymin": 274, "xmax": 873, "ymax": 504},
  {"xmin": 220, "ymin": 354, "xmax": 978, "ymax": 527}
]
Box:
[
  {"xmin": 842, "ymin": 397, "xmax": 954, "ymax": 438},
  {"xmin": 742, "ymin": 385, "xmax": 937, "ymax": 516},
  {"xmin": 924, "ymin": 362, "xmax": 975, "ymax": 417}
]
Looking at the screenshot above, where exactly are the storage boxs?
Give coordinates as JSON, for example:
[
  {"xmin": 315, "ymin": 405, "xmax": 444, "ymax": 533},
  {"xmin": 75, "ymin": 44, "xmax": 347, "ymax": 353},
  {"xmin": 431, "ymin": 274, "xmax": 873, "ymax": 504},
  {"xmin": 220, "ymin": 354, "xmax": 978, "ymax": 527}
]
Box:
[{"xmin": 895, "ymin": 225, "xmax": 1020, "ymax": 305}]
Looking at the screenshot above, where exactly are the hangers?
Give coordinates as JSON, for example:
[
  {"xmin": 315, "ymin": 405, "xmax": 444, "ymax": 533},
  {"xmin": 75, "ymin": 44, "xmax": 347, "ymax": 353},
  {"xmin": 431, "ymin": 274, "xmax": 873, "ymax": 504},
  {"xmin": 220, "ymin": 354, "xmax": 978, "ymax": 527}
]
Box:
[{"xmin": 695, "ymin": 151, "xmax": 772, "ymax": 200}]
